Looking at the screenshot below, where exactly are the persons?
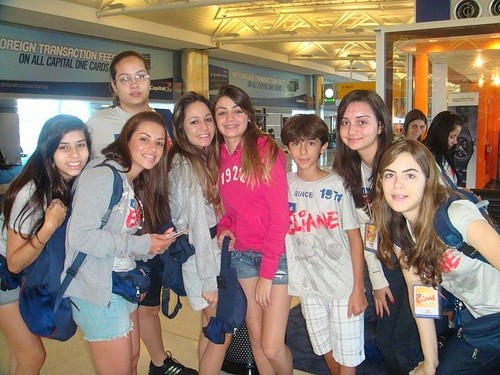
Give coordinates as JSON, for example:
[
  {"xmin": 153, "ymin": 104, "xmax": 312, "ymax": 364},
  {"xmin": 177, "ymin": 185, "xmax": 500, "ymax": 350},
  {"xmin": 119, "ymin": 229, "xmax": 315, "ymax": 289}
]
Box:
[
  {"xmin": 279, "ymin": 114, "xmax": 369, "ymax": 375},
  {"xmin": 0, "ymin": 115, "xmax": 92, "ymax": 375},
  {"xmin": 371, "ymin": 138, "xmax": 500, "ymax": 375},
  {"xmin": 59, "ymin": 109, "xmax": 177, "ymax": 375},
  {"xmin": 83, "ymin": 50, "xmax": 199, "ymax": 375},
  {"xmin": 216, "ymin": 85, "xmax": 293, "ymax": 375},
  {"xmin": 399, "ymin": 109, "xmax": 482, "ymax": 202},
  {"xmin": 335, "ymin": 89, "xmax": 425, "ymax": 375},
  {"xmin": 165, "ymin": 91, "xmax": 248, "ymax": 375}
]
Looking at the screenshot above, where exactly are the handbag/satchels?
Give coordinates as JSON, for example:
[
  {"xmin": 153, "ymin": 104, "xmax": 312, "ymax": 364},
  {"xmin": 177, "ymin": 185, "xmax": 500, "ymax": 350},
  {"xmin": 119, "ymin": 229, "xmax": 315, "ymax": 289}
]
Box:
[
  {"xmin": 109, "ymin": 266, "xmax": 152, "ymax": 304},
  {"xmin": 205, "ymin": 236, "xmax": 249, "ymax": 344},
  {"xmin": 221, "ymin": 320, "xmax": 258, "ymax": 375},
  {"xmin": 158, "ymin": 223, "xmax": 196, "ymax": 320}
]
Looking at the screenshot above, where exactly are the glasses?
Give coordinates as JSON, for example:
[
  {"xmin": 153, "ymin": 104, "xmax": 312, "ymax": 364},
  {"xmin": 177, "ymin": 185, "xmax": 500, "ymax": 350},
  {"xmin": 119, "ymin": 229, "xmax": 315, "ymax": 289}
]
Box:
[{"xmin": 114, "ymin": 73, "xmax": 153, "ymax": 85}]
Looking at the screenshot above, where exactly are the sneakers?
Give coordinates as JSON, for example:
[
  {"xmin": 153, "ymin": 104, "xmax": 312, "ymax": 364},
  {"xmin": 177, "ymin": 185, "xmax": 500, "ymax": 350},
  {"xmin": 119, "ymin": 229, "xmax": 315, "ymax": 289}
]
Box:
[{"xmin": 148, "ymin": 351, "xmax": 204, "ymax": 375}]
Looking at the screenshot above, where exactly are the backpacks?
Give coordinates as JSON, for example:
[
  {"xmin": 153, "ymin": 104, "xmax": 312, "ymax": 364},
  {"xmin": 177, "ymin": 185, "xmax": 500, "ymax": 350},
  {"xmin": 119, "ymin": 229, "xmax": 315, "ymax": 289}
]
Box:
[{"xmin": 17, "ymin": 163, "xmax": 124, "ymax": 344}]
